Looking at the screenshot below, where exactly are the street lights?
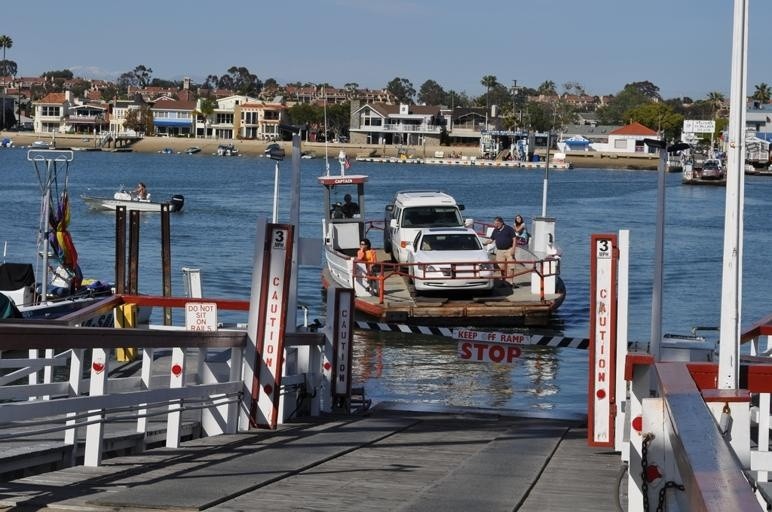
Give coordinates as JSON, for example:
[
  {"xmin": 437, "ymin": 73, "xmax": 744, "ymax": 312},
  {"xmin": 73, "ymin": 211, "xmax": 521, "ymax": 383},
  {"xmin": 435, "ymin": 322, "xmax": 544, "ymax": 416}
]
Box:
[{"xmin": 15, "ymin": 79, "xmax": 23, "ymax": 131}]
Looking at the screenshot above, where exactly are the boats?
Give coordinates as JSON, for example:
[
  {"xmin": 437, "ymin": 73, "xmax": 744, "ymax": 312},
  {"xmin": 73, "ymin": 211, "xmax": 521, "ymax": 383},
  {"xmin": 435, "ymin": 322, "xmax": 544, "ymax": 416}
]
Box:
[
  {"xmin": 321, "ymin": 89, "xmax": 568, "ymax": 325},
  {"xmin": 346, "ymin": 318, "xmax": 563, "ymax": 415},
  {"xmin": 79, "ymin": 182, "xmax": 184, "ymax": 213},
  {"xmin": 668, "ymin": 137, "xmax": 772, "ymax": 185},
  {"xmin": 0, "ymin": 146, "xmax": 157, "ymax": 351}
]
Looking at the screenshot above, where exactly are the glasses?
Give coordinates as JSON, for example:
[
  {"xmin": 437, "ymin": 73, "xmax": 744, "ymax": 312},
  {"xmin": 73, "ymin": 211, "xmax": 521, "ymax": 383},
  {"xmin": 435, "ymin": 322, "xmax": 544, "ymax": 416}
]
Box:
[{"xmin": 360, "ymin": 244, "xmax": 366, "ymax": 245}]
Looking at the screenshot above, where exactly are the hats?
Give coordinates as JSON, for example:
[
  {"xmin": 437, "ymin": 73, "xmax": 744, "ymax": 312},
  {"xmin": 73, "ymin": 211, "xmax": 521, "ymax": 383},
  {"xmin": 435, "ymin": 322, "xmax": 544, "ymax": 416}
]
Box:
[{"xmin": 331, "ymin": 202, "xmax": 342, "ymax": 208}]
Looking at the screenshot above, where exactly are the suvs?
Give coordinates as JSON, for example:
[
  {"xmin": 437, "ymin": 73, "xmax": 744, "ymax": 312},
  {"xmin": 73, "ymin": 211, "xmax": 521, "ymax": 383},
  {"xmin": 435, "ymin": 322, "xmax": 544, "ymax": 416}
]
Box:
[{"xmin": 382, "ymin": 186, "xmax": 467, "ymax": 276}]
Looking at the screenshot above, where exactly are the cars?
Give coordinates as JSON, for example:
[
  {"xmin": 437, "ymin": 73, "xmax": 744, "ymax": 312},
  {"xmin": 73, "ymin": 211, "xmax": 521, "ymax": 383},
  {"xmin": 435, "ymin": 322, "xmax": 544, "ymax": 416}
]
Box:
[
  {"xmin": 407, "ymin": 226, "xmax": 499, "ymax": 293},
  {"xmin": 22, "ymin": 121, "xmax": 34, "ymax": 130}
]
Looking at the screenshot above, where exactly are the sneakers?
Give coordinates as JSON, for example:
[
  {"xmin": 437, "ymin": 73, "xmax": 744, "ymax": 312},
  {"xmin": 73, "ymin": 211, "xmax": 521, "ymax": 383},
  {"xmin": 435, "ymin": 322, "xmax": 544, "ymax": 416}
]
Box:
[
  {"xmin": 502, "ymin": 282, "xmax": 507, "ymax": 287},
  {"xmin": 510, "ymin": 282, "xmax": 516, "ymax": 288}
]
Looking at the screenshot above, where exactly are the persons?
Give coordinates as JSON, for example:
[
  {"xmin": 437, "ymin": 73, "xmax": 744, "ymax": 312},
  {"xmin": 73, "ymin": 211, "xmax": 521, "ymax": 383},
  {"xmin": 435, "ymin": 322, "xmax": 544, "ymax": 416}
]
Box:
[
  {"xmin": 513, "ymin": 215, "xmax": 528, "ymax": 240},
  {"xmin": 482, "ymin": 216, "xmax": 519, "ymax": 288},
  {"xmin": 356, "ymin": 239, "xmax": 377, "ymax": 295},
  {"xmin": 330, "ymin": 194, "xmax": 360, "ymax": 219},
  {"xmin": 136, "ymin": 182, "xmax": 145, "ymax": 199}
]
